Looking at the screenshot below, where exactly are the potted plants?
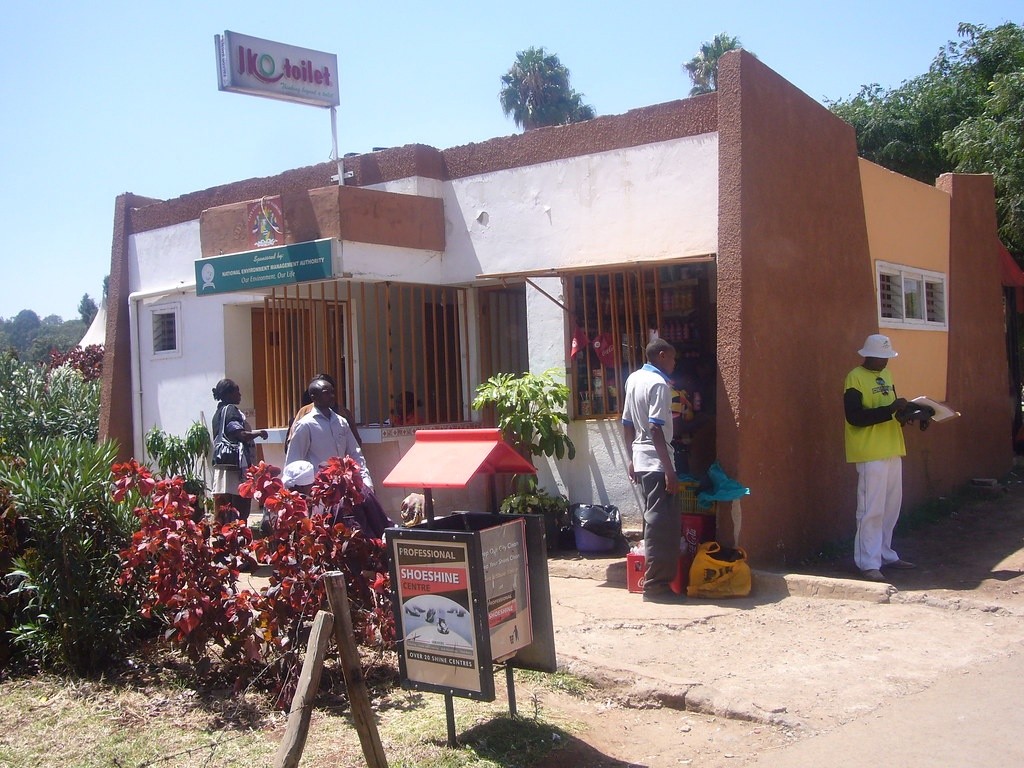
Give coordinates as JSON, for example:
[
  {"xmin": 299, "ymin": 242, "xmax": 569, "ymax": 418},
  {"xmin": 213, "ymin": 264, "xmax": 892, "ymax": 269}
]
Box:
[
  {"xmin": 473, "ymin": 371, "xmax": 576, "ymax": 544},
  {"xmin": 144, "ymin": 422, "xmax": 210, "ymax": 524}
]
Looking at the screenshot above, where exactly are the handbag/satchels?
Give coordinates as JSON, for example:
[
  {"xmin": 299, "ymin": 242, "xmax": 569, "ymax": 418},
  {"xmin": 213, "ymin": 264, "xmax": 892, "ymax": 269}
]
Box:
[
  {"xmin": 212, "ymin": 404, "xmax": 251, "ymax": 471},
  {"xmin": 687, "ymin": 541, "xmax": 751, "ymax": 597}
]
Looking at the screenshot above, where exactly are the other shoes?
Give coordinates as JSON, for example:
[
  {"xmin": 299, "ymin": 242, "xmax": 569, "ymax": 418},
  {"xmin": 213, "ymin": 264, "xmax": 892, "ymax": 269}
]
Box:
[
  {"xmin": 882, "ymin": 559, "xmax": 915, "ymax": 569},
  {"xmin": 859, "ymin": 569, "xmax": 886, "ymax": 581},
  {"xmin": 643, "ymin": 589, "xmax": 688, "ymax": 603}
]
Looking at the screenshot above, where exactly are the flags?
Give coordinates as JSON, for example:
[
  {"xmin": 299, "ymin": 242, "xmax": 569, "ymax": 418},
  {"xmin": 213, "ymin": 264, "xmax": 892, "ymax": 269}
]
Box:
[{"xmin": 571, "ymin": 324, "xmax": 589, "ymax": 360}]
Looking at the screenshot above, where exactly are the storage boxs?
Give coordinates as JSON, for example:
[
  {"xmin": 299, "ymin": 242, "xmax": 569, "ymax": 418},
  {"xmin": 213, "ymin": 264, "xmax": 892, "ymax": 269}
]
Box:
[
  {"xmin": 676, "ymin": 481, "xmax": 717, "ymax": 516},
  {"xmin": 679, "ymin": 513, "xmax": 716, "ymax": 573},
  {"xmin": 627, "ymin": 552, "xmax": 690, "ymax": 594}
]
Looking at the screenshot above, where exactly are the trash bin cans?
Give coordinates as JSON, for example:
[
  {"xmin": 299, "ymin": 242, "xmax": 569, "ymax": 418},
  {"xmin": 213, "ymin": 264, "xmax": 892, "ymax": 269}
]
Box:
[
  {"xmin": 382, "ymin": 429, "xmax": 557, "ymax": 703},
  {"xmin": 573, "ymin": 505, "xmax": 616, "ymax": 550}
]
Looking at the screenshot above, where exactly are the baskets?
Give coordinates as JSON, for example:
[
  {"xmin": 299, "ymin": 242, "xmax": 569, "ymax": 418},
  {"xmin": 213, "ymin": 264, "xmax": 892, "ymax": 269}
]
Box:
[{"xmin": 677, "ymin": 481, "xmax": 716, "ymax": 516}]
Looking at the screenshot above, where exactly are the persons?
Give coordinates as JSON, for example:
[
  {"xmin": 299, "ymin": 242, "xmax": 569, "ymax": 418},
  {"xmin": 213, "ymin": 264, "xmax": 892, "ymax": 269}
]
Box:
[
  {"xmin": 400, "ymin": 493, "xmax": 435, "ymax": 526},
  {"xmin": 211, "ymin": 379, "xmax": 268, "ymax": 526},
  {"xmin": 844, "ymin": 335, "xmax": 935, "ymax": 580},
  {"xmin": 622, "ymin": 339, "xmax": 688, "ymax": 604},
  {"xmin": 284, "ymin": 374, "xmax": 362, "ymax": 455},
  {"xmin": 282, "ymin": 460, "xmax": 315, "ymax": 495},
  {"xmin": 311, "ymin": 462, "xmax": 393, "ymax": 538},
  {"xmin": 284, "ymin": 380, "xmax": 389, "ymax": 537},
  {"xmin": 395, "ymin": 391, "xmax": 425, "ymax": 426}
]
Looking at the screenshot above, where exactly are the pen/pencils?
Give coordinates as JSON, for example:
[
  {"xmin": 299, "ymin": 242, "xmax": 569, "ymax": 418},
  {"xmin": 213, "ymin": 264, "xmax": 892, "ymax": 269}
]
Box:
[{"xmin": 893, "ymin": 384, "xmax": 898, "ymax": 400}]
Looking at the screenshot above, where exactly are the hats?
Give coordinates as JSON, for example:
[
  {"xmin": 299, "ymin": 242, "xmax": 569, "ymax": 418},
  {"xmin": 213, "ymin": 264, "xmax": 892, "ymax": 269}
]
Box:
[
  {"xmin": 858, "ymin": 334, "xmax": 898, "ymax": 358},
  {"xmin": 281, "ymin": 460, "xmax": 315, "ymax": 489}
]
